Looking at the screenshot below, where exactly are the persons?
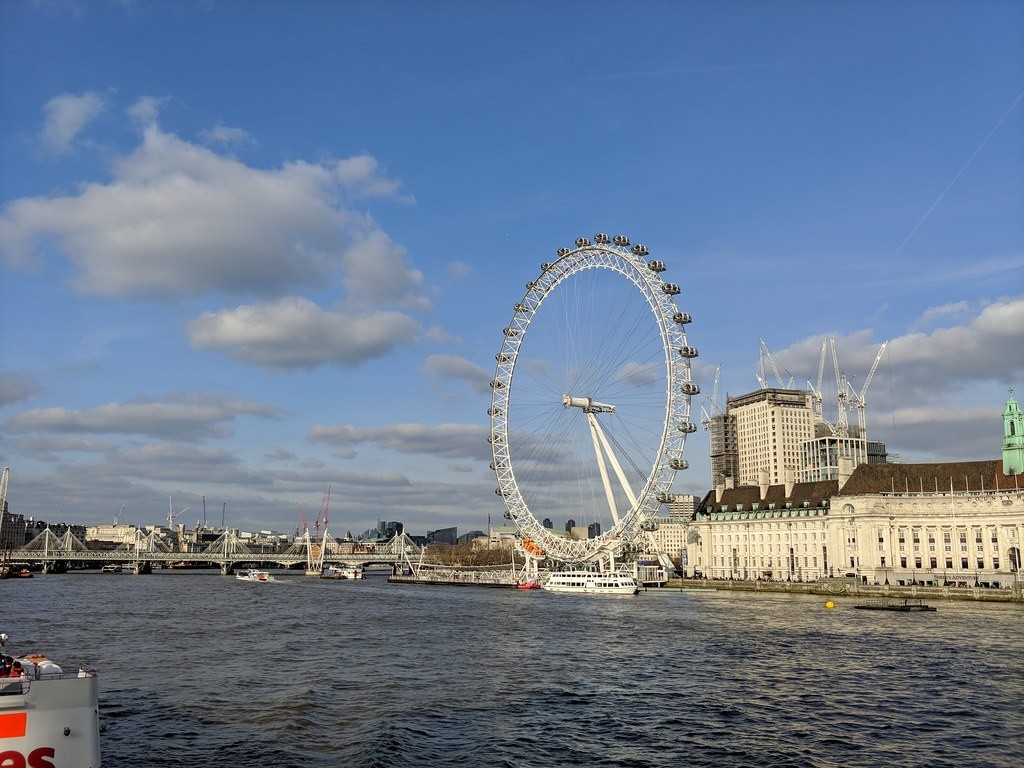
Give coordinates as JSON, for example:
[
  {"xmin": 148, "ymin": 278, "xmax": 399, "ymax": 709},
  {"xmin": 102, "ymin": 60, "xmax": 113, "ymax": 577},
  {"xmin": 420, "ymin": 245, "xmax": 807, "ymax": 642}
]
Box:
[{"xmin": 1, "ymin": 653, "xmax": 24, "ymax": 677}]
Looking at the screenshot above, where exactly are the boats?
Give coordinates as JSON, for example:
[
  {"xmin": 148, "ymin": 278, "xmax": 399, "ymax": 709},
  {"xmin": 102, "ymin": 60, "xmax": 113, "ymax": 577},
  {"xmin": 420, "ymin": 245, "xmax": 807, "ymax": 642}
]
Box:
[
  {"xmin": 11, "ymin": 569, "xmax": 33, "ymax": 578},
  {"xmin": 855, "ymin": 600, "xmax": 937, "ymax": 612},
  {"xmin": 320, "ymin": 562, "xmax": 365, "ymax": 578},
  {"xmin": 543, "ymin": 570, "xmax": 638, "ymax": 594},
  {"xmin": 236, "ymin": 569, "xmax": 271, "ymax": 582},
  {"xmin": 102, "ymin": 564, "xmax": 122, "ymax": 572}
]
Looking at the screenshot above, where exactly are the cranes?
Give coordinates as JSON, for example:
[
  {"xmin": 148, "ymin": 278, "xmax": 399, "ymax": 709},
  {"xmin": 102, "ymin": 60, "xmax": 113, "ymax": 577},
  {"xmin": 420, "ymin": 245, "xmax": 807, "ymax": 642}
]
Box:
[
  {"xmin": 166, "ymin": 496, "xmax": 190, "ymax": 531},
  {"xmin": 323, "ymin": 484, "xmax": 330, "ymax": 528},
  {"xmin": 315, "ymin": 499, "xmax": 325, "ymax": 542},
  {"xmin": 297, "ymin": 501, "xmax": 307, "ymax": 538},
  {"xmin": 114, "ymin": 504, "xmax": 125, "ymax": 525}
]
